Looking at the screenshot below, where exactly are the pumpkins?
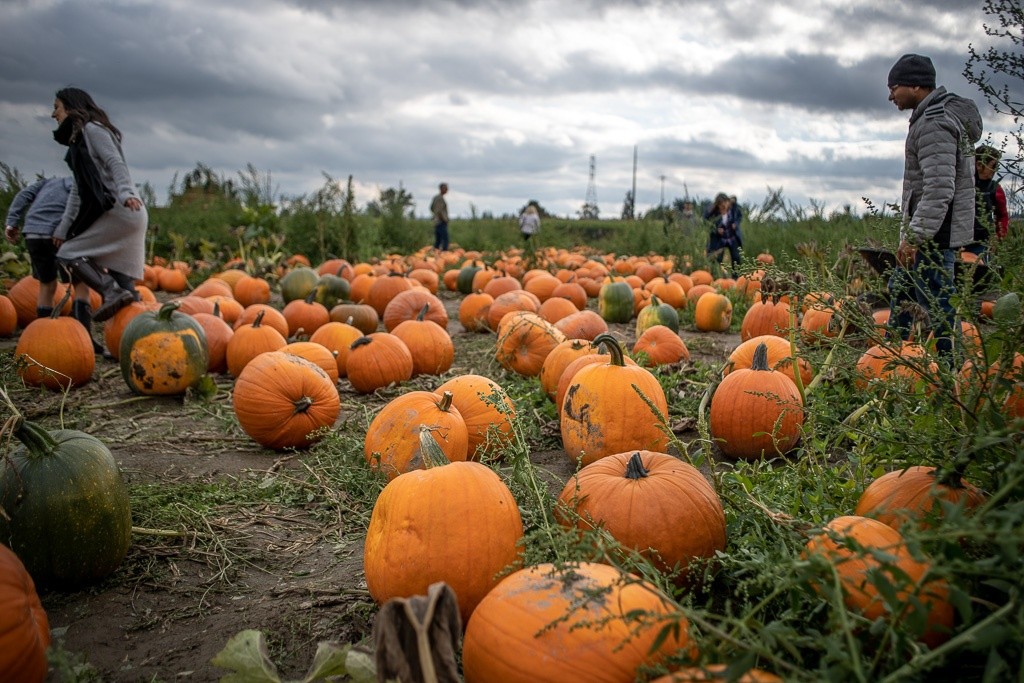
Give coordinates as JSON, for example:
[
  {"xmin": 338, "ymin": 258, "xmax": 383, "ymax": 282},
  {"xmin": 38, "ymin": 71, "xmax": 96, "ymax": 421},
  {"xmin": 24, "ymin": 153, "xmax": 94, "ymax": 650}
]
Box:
[
  {"xmin": 364, "ymin": 389, "xmax": 468, "ymax": 482},
  {"xmin": 0, "ymin": 543, "xmax": 52, "ymax": 683},
  {"xmin": 854, "ymin": 462, "xmax": 991, "ymax": 535},
  {"xmin": 459, "ymin": 561, "xmax": 701, "ymax": 683},
  {"xmin": 770, "ymin": 511, "xmax": 956, "ymax": 649},
  {"xmin": 0, "ymin": 416, "xmax": 133, "ymax": 593},
  {"xmin": 0, "ymin": 241, "xmax": 1024, "ymax": 459},
  {"xmin": 553, "ymin": 449, "xmax": 726, "ymax": 591},
  {"xmin": 641, "ymin": 664, "xmax": 787, "ymax": 683},
  {"xmin": 363, "ymin": 430, "xmax": 529, "ymax": 627}
]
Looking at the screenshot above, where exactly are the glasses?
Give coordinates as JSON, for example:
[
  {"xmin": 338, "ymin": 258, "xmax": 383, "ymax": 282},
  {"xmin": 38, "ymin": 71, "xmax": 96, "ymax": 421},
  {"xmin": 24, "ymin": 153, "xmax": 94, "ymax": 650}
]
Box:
[
  {"xmin": 889, "ymin": 85, "xmax": 898, "ymax": 94},
  {"xmin": 991, "ymin": 164, "xmax": 997, "ymax": 169}
]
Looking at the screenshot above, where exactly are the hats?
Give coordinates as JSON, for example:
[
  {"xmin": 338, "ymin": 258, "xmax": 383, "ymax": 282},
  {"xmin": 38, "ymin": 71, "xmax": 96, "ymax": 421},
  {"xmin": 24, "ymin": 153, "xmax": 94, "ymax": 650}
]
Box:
[{"xmin": 888, "ymin": 54, "xmax": 936, "ymax": 89}]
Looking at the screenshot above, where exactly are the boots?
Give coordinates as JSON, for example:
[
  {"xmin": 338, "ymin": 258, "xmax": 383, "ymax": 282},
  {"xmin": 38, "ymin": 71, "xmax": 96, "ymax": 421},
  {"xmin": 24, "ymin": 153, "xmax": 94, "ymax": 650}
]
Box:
[
  {"xmin": 70, "ymin": 299, "xmax": 103, "ymax": 355},
  {"xmin": 36, "ymin": 307, "xmax": 53, "ymax": 318},
  {"xmin": 67, "ymin": 257, "xmax": 133, "ymax": 323}
]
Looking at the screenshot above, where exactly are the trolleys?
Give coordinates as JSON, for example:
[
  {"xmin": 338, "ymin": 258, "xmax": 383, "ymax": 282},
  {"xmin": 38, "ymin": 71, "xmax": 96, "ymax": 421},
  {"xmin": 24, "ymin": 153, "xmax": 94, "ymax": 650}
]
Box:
[{"xmin": 851, "ymin": 246, "xmax": 1006, "ymax": 302}]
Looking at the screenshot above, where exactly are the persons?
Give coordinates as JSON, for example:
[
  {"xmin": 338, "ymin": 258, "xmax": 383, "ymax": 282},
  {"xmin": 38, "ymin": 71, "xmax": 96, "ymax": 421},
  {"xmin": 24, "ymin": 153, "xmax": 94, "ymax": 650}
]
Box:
[
  {"xmin": 53, "ymin": 88, "xmax": 148, "ymax": 321},
  {"xmin": 702, "ymin": 192, "xmax": 745, "ymax": 280},
  {"xmin": 5, "ymin": 176, "xmax": 108, "ymax": 354},
  {"xmin": 430, "ymin": 183, "xmax": 450, "ymax": 251},
  {"xmin": 973, "ymin": 146, "xmax": 1008, "ymax": 263},
  {"xmin": 519, "ymin": 205, "xmax": 542, "ymax": 240},
  {"xmin": 888, "ymin": 53, "xmax": 983, "ymax": 415}
]
